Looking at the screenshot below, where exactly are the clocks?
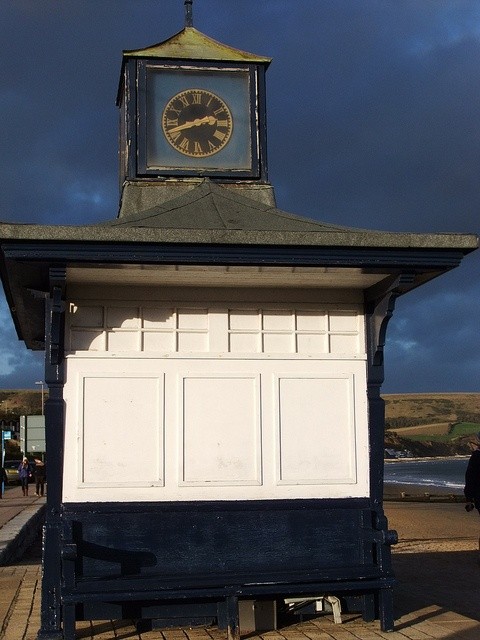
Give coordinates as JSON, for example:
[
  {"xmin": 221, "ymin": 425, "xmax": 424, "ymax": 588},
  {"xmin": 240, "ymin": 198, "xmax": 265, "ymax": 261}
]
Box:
[{"xmin": 136, "ymin": 58, "xmax": 260, "ymax": 179}]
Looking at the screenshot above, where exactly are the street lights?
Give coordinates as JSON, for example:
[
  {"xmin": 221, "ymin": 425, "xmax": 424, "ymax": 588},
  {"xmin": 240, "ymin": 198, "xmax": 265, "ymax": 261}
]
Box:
[
  {"xmin": 2, "ymin": 401, "xmax": 7, "ymax": 414},
  {"xmin": 12, "ymin": 411, "xmax": 14, "ymax": 439},
  {"xmin": 35, "ymin": 381, "xmax": 45, "ymax": 462}
]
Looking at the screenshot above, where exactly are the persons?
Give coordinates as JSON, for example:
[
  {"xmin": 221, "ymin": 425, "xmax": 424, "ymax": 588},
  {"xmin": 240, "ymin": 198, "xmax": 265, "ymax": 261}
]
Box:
[
  {"xmin": 1, "ymin": 466, "xmax": 8, "ymax": 496},
  {"xmin": 17, "ymin": 457, "xmax": 33, "ymax": 496},
  {"xmin": 32, "ymin": 458, "xmax": 47, "ymax": 496}
]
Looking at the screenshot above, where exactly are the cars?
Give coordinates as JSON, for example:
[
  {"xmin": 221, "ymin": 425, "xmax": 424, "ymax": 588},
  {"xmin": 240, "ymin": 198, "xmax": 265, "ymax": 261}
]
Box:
[{"xmin": 5, "ymin": 460, "xmax": 34, "ymax": 485}]
